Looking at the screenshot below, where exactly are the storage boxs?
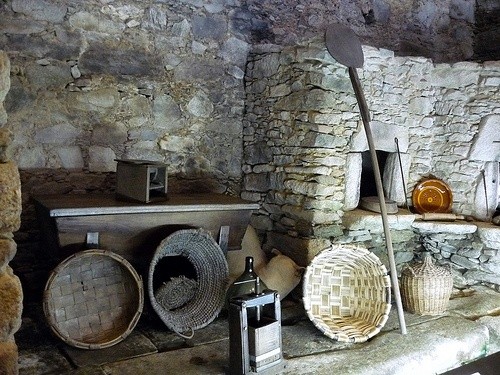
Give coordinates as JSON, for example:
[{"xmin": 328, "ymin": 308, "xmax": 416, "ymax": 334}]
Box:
[{"xmin": 112, "ymin": 156, "xmax": 170, "ymax": 205}]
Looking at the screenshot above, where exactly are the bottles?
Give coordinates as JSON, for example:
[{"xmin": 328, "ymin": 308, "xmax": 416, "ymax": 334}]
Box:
[{"xmin": 224, "ymin": 255, "xmax": 270, "ymax": 321}]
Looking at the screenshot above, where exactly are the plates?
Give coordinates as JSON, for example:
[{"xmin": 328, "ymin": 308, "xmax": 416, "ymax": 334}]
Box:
[{"xmin": 411, "ymin": 177, "xmax": 454, "ymax": 215}]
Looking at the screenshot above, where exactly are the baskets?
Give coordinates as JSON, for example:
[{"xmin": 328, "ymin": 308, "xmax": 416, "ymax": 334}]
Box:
[
  {"xmin": 148, "ymin": 228, "xmax": 229, "ymax": 340},
  {"xmin": 40, "ymin": 248, "xmax": 143, "ymax": 350},
  {"xmin": 304, "ymin": 243, "xmax": 392, "ymax": 343}
]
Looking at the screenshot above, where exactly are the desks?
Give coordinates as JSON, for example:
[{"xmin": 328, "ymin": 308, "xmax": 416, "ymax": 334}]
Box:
[{"xmin": 31, "ymin": 190, "xmax": 260, "ymax": 262}]
